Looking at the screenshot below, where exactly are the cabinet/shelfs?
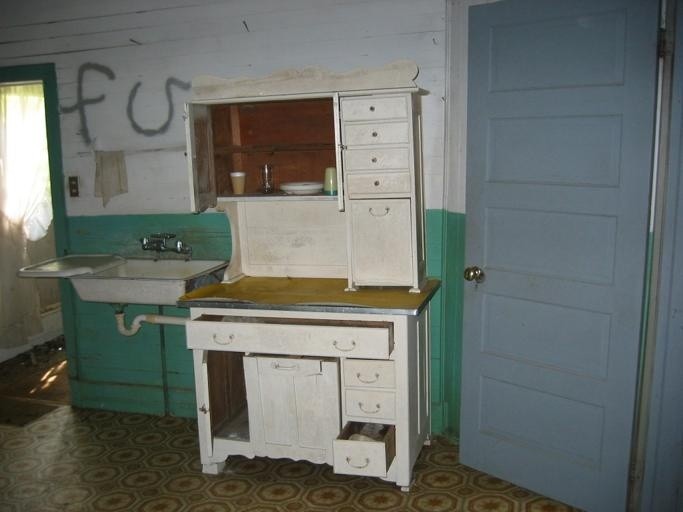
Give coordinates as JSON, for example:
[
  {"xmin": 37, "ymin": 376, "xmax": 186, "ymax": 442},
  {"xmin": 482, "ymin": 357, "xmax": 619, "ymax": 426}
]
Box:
[
  {"xmin": 186, "ymin": 299, "xmax": 431, "ymax": 360},
  {"xmin": 344, "ymin": 193, "xmax": 428, "ymax": 294},
  {"xmin": 339, "ymin": 87, "xmax": 430, "ymax": 199},
  {"xmin": 193, "ymin": 350, "xmax": 265, "ymax": 475},
  {"xmin": 332, "ymin": 357, "xmax": 433, "ymax": 493},
  {"xmin": 185, "ymin": 91, "xmax": 356, "ymax": 291},
  {"xmin": 254, "ymin": 356, "xmax": 341, "ymax": 466}
]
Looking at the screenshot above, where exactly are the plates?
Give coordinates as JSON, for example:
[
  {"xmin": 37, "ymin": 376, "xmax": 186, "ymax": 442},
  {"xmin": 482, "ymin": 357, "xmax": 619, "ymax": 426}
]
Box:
[{"xmin": 280, "ymin": 181, "xmax": 324, "ymax": 195}]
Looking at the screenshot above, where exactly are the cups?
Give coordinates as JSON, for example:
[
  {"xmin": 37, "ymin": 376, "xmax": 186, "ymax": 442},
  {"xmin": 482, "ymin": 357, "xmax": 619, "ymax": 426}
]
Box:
[
  {"xmin": 231, "ymin": 171, "xmax": 246, "ymax": 195},
  {"xmin": 323, "ymin": 166, "xmax": 337, "ymax": 196}
]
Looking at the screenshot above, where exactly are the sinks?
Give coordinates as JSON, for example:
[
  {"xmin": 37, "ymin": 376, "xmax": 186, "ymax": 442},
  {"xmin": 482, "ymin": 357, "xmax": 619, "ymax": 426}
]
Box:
[{"xmin": 152, "ymin": 232, "xmax": 173, "ymax": 252}]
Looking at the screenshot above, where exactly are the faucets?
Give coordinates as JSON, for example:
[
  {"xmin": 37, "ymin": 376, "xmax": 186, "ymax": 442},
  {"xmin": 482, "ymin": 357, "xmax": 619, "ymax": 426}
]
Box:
[{"xmin": 71, "ymin": 256, "xmax": 228, "ymax": 306}]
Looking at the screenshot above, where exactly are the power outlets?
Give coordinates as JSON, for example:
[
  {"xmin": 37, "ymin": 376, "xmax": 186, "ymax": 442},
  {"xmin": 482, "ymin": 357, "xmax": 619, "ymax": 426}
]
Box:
[{"xmin": 69, "ymin": 176, "xmax": 79, "ymax": 198}]
